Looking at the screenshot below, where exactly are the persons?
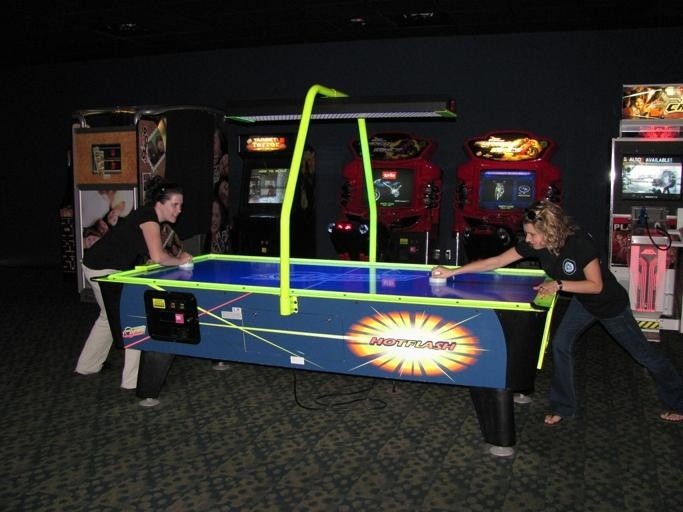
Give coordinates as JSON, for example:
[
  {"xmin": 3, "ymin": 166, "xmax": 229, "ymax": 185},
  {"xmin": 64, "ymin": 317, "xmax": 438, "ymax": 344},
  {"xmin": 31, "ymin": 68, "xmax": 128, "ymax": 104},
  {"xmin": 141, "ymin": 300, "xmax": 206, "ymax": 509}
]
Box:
[
  {"xmin": 70, "ymin": 174, "xmax": 193, "ymax": 392},
  {"xmin": 210, "ymin": 198, "xmax": 229, "ymax": 259},
  {"xmin": 81, "ymin": 189, "xmax": 110, "ymax": 233},
  {"xmin": 212, "ymin": 174, "xmax": 228, "ymax": 211},
  {"xmin": 106, "ymin": 190, "xmax": 133, "ymax": 228},
  {"xmin": 429, "ymin": 197, "xmax": 682, "ymax": 427},
  {"xmin": 213, "ymin": 127, "xmax": 228, "ymax": 184},
  {"xmin": 82, "ymin": 231, "xmax": 102, "ymax": 251},
  {"xmin": 156, "ymin": 136, "xmax": 163, "ymax": 153}
]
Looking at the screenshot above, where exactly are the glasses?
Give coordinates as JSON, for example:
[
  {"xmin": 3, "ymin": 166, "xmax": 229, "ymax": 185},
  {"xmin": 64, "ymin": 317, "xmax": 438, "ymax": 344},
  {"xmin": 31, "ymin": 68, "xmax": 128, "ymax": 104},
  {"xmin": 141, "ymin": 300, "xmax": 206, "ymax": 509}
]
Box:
[{"xmin": 524, "ymin": 207, "xmax": 544, "ymax": 223}]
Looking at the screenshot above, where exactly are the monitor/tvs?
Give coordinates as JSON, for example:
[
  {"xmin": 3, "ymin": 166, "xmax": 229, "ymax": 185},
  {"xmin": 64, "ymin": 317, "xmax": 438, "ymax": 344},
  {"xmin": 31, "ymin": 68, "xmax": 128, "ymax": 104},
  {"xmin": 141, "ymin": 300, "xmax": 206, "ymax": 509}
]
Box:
[
  {"xmin": 362, "ymin": 168, "xmax": 413, "ymax": 207},
  {"xmin": 478, "ymin": 169, "xmax": 537, "ymax": 211},
  {"xmin": 617, "ymin": 152, "xmax": 683, "ymax": 203},
  {"xmin": 246, "ymin": 166, "xmax": 290, "ymax": 205}
]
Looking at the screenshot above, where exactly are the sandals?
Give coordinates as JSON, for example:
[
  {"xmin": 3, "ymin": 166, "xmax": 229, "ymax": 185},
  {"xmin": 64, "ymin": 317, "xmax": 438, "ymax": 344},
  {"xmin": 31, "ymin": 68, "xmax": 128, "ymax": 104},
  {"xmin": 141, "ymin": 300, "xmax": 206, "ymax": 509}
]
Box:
[
  {"xmin": 658, "ymin": 408, "xmax": 683, "ymax": 426},
  {"xmin": 543, "ymin": 411, "xmax": 576, "ymax": 428}
]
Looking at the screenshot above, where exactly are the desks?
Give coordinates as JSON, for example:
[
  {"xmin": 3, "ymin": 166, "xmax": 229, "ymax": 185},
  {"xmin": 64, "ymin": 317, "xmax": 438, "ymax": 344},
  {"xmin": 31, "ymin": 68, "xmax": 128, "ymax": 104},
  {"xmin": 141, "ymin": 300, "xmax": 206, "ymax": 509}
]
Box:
[{"xmin": 90, "ymin": 253, "xmax": 558, "ymax": 457}]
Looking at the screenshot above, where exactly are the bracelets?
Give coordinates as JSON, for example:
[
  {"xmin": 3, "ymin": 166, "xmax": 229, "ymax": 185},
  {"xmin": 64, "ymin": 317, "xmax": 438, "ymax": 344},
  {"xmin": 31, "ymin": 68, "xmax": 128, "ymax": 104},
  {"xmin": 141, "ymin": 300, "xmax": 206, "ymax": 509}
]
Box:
[{"xmin": 555, "ymin": 280, "xmax": 563, "ymax": 290}]
